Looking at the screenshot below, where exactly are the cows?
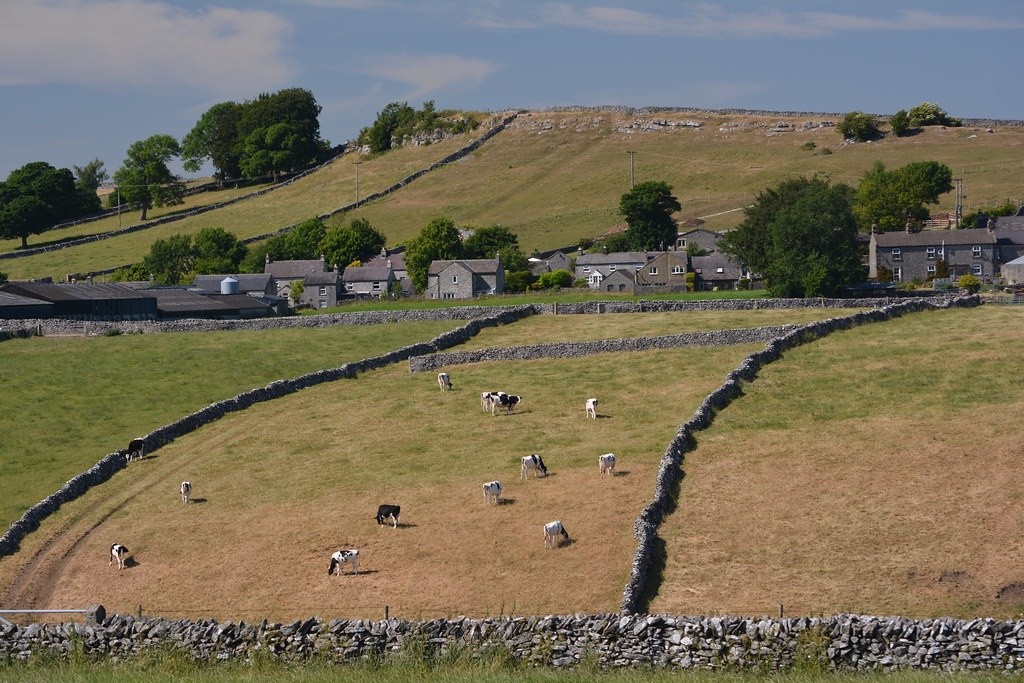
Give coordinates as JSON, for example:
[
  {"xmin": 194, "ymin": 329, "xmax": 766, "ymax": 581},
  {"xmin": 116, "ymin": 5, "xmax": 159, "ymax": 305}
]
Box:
[
  {"xmin": 598, "ymin": 452, "xmax": 616, "ymax": 480},
  {"xmin": 481, "ymin": 391, "xmax": 507, "ymax": 412},
  {"xmin": 437, "ymin": 372, "xmax": 454, "ymax": 392},
  {"xmin": 482, "ymin": 480, "xmax": 502, "ymax": 506},
  {"xmin": 543, "ymin": 520, "xmax": 569, "ymax": 549},
  {"xmin": 327, "ymin": 549, "xmax": 360, "ymax": 578},
  {"xmin": 123, "ymin": 437, "xmax": 145, "ymax": 462},
  {"xmin": 109, "ymin": 542, "xmax": 129, "ymax": 571},
  {"xmin": 585, "ymin": 398, "xmax": 598, "ymax": 421},
  {"xmin": 373, "ymin": 504, "xmax": 401, "ymax": 530},
  {"xmin": 520, "ymin": 454, "xmax": 548, "ymax": 480},
  {"xmin": 180, "ymin": 480, "xmax": 193, "ymax": 504},
  {"xmin": 489, "ymin": 395, "xmax": 523, "ymax": 416}
]
